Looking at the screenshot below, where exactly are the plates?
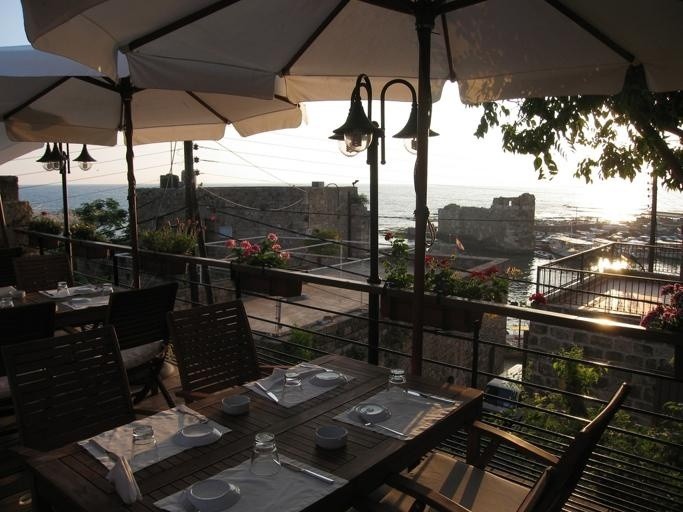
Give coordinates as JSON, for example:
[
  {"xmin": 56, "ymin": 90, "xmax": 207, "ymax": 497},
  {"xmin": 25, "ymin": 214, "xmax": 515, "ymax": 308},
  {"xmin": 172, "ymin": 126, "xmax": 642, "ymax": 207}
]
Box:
[
  {"xmin": 73, "ymin": 287, "xmax": 95, "ymax": 294},
  {"xmin": 309, "ymin": 371, "xmax": 349, "ymax": 387},
  {"xmin": 175, "ymin": 423, "xmax": 222, "ymax": 448},
  {"xmin": 69, "ymin": 298, "xmax": 90, "ymax": 303},
  {"xmin": 180, "ymin": 478, "xmax": 241, "ymax": 512},
  {"xmin": 346, "ymin": 402, "xmax": 392, "ymax": 424}
]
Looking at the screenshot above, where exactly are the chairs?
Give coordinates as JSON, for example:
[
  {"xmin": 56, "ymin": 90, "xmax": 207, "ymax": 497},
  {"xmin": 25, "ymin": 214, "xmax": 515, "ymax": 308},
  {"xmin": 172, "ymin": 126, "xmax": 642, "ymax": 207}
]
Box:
[
  {"xmin": 384, "ymin": 380, "xmax": 630, "ymax": 511},
  {"xmin": 167, "ymin": 299, "xmax": 285, "ymax": 410},
  {"xmin": 11, "ymin": 253, "xmax": 75, "ymax": 293},
  {"xmin": 0, "ymin": 300, "xmax": 62, "ymax": 434},
  {"xmin": 106, "ymin": 281, "xmax": 180, "ymax": 409},
  {"xmin": 0, "ymin": 324, "xmax": 173, "ymax": 460}
]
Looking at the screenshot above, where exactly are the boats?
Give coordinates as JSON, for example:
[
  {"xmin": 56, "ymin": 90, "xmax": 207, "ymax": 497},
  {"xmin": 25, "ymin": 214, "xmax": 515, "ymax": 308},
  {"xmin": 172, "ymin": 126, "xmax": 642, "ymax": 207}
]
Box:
[{"xmin": 536, "ymin": 225, "xmax": 682, "ymax": 262}]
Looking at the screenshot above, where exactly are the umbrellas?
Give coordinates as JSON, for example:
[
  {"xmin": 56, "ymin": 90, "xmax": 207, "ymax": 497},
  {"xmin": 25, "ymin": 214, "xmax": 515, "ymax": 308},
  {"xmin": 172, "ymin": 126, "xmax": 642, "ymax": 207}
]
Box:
[
  {"xmin": 23, "ymin": 2, "xmax": 682, "ymax": 374},
  {"xmin": 1, "ymin": 43, "xmax": 304, "ymax": 291}
]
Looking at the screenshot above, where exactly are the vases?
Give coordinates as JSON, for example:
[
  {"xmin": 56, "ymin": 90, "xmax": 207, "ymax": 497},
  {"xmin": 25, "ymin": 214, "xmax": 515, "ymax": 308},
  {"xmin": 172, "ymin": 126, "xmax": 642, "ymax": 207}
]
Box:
[
  {"xmin": 230, "ymin": 263, "xmax": 302, "ymax": 297},
  {"xmin": 379, "ymin": 289, "xmax": 484, "ymax": 333},
  {"xmin": 140, "ymin": 250, "xmax": 191, "ymax": 277}
]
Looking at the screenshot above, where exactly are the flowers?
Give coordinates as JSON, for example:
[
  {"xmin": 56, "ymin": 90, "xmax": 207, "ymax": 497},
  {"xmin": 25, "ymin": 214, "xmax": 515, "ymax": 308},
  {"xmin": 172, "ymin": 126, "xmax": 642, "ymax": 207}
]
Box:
[
  {"xmin": 224, "ymin": 232, "xmax": 291, "ymax": 270},
  {"xmin": 383, "ymin": 231, "xmax": 549, "ymax": 307},
  {"xmin": 137, "ymin": 217, "xmax": 202, "ymax": 256},
  {"xmin": 639, "ymin": 282, "xmax": 683, "ymax": 330}
]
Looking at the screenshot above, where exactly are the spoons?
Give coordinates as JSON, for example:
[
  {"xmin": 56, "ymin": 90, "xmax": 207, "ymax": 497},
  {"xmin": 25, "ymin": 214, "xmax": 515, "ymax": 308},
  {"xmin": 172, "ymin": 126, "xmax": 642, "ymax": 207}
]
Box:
[{"xmin": 364, "ymin": 421, "xmax": 409, "ymax": 438}]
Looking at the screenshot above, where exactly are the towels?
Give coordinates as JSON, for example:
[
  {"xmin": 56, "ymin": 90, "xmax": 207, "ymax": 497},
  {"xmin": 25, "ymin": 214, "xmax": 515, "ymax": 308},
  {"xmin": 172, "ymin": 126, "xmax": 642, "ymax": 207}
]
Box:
[{"xmin": 107, "ymin": 456, "xmax": 144, "ymax": 505}]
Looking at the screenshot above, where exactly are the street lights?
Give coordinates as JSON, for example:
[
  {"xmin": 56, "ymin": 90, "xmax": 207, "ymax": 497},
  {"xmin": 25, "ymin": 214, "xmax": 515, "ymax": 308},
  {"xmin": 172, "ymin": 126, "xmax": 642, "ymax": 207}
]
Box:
[
  {"xmin": 36, "ymin": 131, "xmax": 97, "ymax": 287},
  {"xmin": 325, "ymin": 71, "xmax": 438, "ymax": 372}
]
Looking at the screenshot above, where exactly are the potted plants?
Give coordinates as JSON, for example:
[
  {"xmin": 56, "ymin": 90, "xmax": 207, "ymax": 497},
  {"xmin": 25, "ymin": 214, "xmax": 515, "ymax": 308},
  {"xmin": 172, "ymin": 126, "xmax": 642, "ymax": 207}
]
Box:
[
  {"xmin": 70, "ymin": 195, "xmax": 128, "ymax": 257},
  {"xmin": 42, "ymin": 218, "xmax": 63, "ymax": 247}
]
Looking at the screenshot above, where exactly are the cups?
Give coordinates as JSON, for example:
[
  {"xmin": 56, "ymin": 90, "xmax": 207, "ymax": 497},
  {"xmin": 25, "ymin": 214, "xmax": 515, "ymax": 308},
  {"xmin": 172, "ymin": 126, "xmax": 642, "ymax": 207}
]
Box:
[
  {"xmin": 0, "ymin": 296, "xmax": 14, "ymax": 307},
  {"xmin": 386, "ymin": 369, "xmax": 408, "ymax": 404},
  {"xmin": 250, "ymin": 432, "xmax": 281, "ymax": 476},
  {"xmin": 281, "ymin": 371, "xmax": 304, "ymax": 404},
  {"xmin": 102, "ymin": 283, "xmax": 114, "ymax": 298},
  {"xmin": 131, "ymin": 425, "xmax": 159, "ymax": 468},
  {"xmin": 56, "ymin": 281, "xmax": 70, "ymax": 298}
]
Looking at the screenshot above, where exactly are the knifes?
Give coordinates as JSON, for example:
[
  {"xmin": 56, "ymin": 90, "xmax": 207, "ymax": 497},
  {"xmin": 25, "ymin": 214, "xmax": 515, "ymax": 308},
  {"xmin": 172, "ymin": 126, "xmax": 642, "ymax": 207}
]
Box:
[
  {"xmin": 88, "ymin": 438, "xmax": 120, "ymax": 462},
  {"xmin": 254, "ymin": 380, "xmax": 278, "ymax": 403},
  {"xmin": 272, "ymin": 457, "xmax": 335, "ymax": 484},
  {"xmin": 403, "ymin": 389, "xmax": 457, "ymax": 405}
]
